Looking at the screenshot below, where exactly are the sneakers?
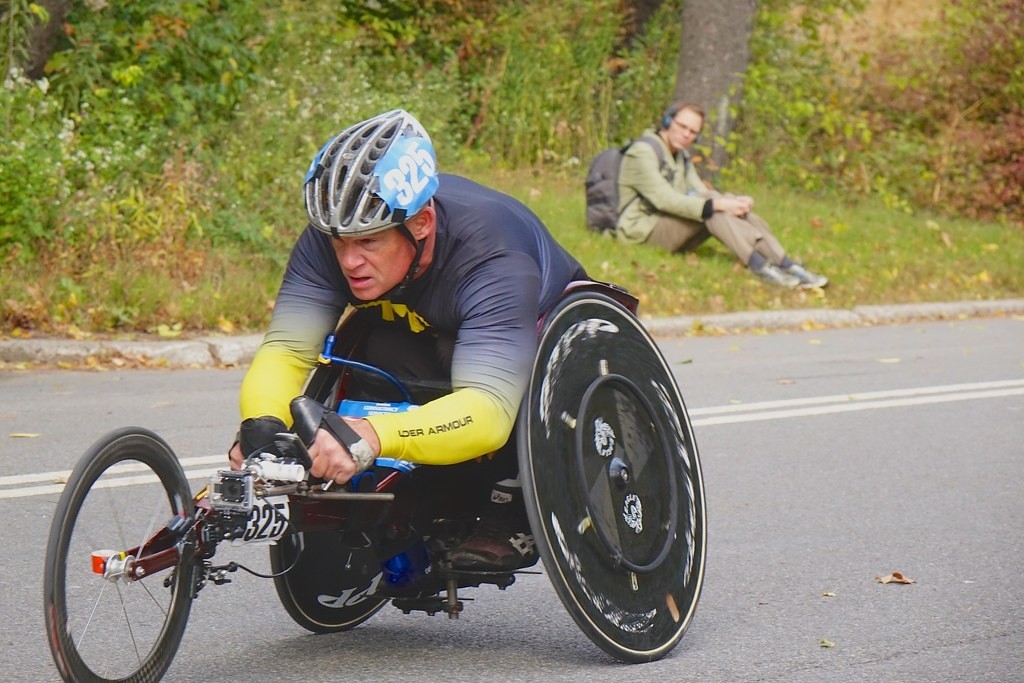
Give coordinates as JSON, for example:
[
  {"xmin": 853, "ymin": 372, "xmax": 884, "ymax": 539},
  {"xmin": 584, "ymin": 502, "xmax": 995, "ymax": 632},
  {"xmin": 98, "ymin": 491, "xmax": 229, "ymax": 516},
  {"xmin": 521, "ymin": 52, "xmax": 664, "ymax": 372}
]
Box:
[
  {"xmin": 423, "ymin": 510, "xmax": 539, "ymax": 572},
  {"xmin": 751, "ymin": 261, "xmax": 830, "ymax": 292}
]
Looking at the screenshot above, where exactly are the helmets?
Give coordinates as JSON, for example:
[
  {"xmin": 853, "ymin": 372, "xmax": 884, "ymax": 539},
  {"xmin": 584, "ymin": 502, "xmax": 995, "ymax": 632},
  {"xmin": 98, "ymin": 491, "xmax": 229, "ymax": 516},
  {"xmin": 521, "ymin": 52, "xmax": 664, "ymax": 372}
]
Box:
[{"xmin": 301, "ymin": 107, "xmax": 440, "ymax": 239}]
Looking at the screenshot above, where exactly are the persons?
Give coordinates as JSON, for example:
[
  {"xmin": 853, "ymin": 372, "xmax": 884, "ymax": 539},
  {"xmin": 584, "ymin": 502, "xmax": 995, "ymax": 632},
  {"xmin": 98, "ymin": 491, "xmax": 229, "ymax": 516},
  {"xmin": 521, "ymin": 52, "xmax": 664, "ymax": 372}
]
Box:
[
  {"xmin": 615, "ymin": 103, "xmax": 832, "ymax": 292},
  {"xmin": 228, "ymin": 109, "xmax": 590, "ymax": 573}
]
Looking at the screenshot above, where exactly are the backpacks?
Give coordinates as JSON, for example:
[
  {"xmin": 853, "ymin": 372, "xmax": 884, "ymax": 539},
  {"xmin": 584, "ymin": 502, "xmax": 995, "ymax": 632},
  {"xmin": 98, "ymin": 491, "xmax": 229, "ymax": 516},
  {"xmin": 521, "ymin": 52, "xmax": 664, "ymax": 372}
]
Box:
[{"xmin": 585, "ymin": 136, "xmax": 694, "ymax": 235}]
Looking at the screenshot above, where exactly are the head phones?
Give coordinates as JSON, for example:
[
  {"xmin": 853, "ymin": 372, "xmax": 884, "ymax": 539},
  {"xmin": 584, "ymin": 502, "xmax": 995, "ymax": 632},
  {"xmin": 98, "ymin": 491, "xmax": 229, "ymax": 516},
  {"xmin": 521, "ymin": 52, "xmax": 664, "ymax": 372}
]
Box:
[{"xmin": 663, "ymin": 104, "xmax": 702, "ymax": 143}]
